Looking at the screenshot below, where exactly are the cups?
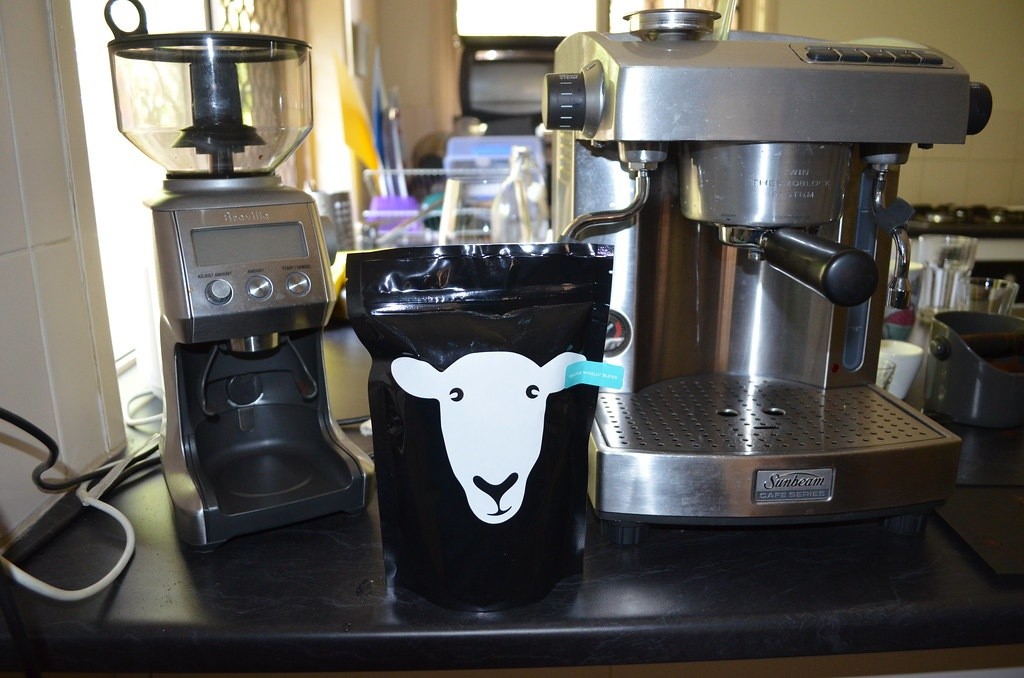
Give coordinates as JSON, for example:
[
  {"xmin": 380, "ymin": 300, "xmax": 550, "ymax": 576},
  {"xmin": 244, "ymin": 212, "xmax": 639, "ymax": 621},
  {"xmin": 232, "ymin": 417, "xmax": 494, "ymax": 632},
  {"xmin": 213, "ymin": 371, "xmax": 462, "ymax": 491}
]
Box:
[
  {"xmin": 875, "ymin": 339, "xmax": 925, "ymax": 400},
  {"xmin": 954, "ymin": 276, "xmax": 1018, "ymax": 316},
  {"xmin": 917, "ymin": 233, "xmax": 978, "ymax": 324}
]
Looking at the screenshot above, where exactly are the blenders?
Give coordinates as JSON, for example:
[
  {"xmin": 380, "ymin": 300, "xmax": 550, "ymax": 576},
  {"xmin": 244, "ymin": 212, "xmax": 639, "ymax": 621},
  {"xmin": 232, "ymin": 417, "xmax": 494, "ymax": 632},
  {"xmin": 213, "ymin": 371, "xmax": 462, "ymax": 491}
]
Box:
[{"xmin": 105, "ymin": 29, "xmax": 375, "ymax": 558}]
